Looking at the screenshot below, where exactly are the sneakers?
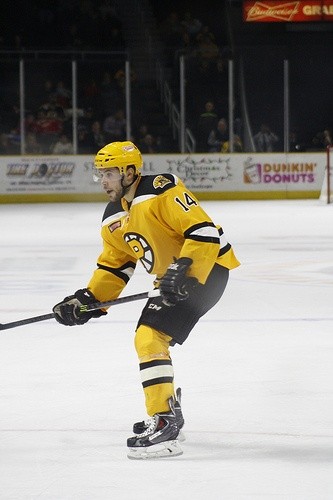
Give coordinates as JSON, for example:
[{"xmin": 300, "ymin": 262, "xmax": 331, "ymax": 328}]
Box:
[
  {"xmin": 125, "ymin": 397, "xmax": 184, "ymax": 460},
  {"xmin": 131, "ymin": 388, "xmax": 186, "ymax": 444}
]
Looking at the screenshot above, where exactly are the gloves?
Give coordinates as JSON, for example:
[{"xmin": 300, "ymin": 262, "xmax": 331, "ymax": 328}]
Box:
[
  {"xmin": 52, "ymin": 288, "xmax": 100, "ymax": 327},
  {"xmin": 159, "ymin": 257, "xmax": 200, "ymax": 305}
]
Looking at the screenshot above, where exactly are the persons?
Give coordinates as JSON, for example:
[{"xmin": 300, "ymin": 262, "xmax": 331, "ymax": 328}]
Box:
[
  {"xmin": 52, "ymin": 141, "xmax": 238, "ymax": 460},
  {"xmin": 0, "ymin": 0, "xmax": 333, "ymax": 156}
]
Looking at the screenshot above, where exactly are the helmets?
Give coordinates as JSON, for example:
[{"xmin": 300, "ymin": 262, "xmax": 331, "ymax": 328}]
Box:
[{"xmin": 95, "ymin": 141, "xmax": 143, "ymax": 177}]
